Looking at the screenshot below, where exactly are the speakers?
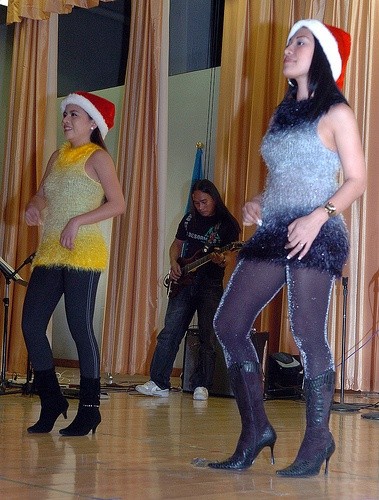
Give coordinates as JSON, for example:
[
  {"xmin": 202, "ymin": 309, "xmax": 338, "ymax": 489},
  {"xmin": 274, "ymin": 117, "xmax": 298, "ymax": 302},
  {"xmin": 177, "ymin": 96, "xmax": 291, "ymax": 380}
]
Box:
[{"xmin": 182, "ymin": 328, "xmax": 266, "ymax": 399}]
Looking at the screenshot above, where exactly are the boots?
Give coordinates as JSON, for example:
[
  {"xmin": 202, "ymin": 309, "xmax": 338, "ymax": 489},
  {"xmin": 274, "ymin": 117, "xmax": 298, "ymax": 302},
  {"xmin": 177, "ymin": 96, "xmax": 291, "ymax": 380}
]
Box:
[
  {"xmin": 208, "ymin": 359, "xmax": 277, "ymax": 471},
  {"xmin": 276, "ymin": 369, "xmax": 335, "ymax": 477},
  {"xmin": 58, "ymin": 376, "xmax": 102, "ymax": 436},
  {"xmin": 27, "ymin": 366, "xmax": 69, "ymax": 434}
]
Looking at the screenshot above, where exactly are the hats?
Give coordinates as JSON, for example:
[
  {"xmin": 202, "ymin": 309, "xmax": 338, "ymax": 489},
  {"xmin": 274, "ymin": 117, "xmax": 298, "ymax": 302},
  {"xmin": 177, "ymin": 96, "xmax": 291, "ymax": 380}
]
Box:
[
  {"xmin": 286, "ymin": 18, "xmax": 352, "ymax": 92},
  {"xmin": 60, "ymin": 90, "xmax": 116, "ymax": 141}
]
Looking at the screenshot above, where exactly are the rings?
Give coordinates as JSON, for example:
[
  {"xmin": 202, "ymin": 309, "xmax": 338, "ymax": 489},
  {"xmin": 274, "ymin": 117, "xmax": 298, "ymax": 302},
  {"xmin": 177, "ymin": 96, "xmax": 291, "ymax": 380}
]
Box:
[{"xmin": 299, "ymin": 243, "xmax": 304, "ymax": 247}]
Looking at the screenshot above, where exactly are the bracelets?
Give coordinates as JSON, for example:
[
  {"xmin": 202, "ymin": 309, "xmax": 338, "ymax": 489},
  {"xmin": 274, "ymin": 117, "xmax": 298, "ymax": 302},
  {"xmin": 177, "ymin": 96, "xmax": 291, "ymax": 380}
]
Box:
[
  {"xmin": 321, "ymin": 201, "xmax": 337, "ymax": 218},
  {"xmin": 219, "ymin": 255, "xmax": 226, "ymax": 265}
]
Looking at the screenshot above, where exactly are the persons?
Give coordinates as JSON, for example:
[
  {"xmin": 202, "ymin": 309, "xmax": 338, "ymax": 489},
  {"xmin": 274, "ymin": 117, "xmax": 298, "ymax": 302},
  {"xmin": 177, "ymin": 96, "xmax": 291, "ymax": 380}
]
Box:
[
  {"xmin": 135, "ymin": 179, "xmax": 241, "ymax": 401},
  {"xmin": 21, "ymin": 91, "xmax": 126, "ymax": 436},
  {"xmin": 206, "ymin": 19, "xmax": 366, "ymax": 478}
]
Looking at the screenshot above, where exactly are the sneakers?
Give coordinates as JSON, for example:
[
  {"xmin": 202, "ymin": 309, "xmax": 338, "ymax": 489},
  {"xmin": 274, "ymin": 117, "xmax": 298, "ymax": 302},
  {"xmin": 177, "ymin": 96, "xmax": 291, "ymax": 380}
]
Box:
[
  {"xmin": 135, "ymin": 381, "xmax": 170, "ymax": 397},
  {"xmin": 192, "ymin": 386, "xmax": 208, "ymax": 400}
]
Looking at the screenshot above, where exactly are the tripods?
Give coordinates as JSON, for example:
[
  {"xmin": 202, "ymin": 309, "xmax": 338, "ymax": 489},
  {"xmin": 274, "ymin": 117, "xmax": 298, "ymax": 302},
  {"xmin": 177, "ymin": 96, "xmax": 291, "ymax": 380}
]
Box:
[{"xmin": 0, "ymin": 355, "xmax": 76, "ymax": 397}]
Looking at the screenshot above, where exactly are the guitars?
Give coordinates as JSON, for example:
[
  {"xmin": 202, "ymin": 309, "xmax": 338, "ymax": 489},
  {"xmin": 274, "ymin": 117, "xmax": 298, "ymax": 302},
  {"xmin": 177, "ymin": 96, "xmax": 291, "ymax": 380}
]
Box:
[{"xmin": 166, "ymin": 239, "xmax": 246, "ymax": 299}]
